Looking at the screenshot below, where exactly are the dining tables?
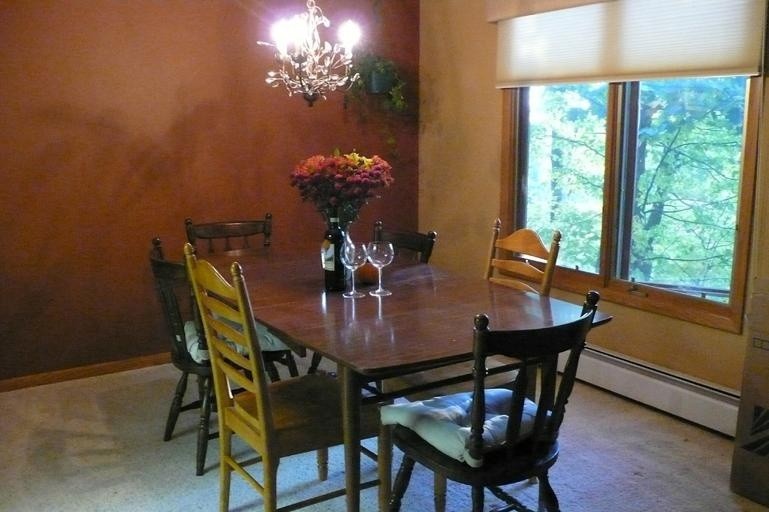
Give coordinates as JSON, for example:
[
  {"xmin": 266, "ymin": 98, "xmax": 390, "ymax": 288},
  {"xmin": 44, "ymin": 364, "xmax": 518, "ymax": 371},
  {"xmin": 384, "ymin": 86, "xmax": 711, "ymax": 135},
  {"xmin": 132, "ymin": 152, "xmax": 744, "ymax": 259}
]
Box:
[{"xmin": 344, "ymin": 53, "xmax": 410, "ymax": 114}]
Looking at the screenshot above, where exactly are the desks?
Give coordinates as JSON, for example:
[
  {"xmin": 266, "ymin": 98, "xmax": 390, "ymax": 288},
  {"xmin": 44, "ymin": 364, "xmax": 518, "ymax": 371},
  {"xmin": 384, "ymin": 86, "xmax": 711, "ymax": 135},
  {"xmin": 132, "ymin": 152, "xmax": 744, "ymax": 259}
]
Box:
[{"xmin": 180, "ymin": 245, "xmax": 613, "ymax": 510}]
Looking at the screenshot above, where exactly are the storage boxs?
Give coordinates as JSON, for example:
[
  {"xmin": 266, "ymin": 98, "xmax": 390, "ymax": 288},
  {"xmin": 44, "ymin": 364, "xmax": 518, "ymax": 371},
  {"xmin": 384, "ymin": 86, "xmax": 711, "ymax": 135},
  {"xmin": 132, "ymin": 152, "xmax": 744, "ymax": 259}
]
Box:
[{"xmin": 729, "ymin": 292, "xmax": 768, "ymax": 506}]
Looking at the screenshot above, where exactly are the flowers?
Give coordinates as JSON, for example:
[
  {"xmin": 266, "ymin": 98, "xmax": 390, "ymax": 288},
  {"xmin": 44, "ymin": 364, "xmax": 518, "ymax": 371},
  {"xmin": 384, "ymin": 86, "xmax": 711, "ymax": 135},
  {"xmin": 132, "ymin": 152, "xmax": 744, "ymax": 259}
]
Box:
[{"xmin": 292, "ymin": 150, "xmax": 394, "ymax": 231}]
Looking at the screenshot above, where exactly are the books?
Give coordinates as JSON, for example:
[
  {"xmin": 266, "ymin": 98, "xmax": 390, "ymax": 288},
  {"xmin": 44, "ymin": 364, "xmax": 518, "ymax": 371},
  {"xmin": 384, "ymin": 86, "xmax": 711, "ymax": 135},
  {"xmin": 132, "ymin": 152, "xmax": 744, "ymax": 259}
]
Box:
[{"xmin": 729, "ymin": 292, "xmax": 768, "ymax": 506}]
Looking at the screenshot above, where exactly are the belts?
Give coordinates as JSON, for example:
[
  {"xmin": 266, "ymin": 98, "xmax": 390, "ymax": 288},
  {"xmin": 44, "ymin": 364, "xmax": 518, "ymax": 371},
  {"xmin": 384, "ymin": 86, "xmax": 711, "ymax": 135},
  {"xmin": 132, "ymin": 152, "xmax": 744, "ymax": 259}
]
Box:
[
  {"xmin": 340, "ymin": 241, "xmax": 368, "ymax": 299},
  {"xmin": 366, "ymin": 240, "xmax": 395, "ymax": 298},
  {"xmin": 342, "ymin": 300, "xmax": 396, "ymax": 356}
]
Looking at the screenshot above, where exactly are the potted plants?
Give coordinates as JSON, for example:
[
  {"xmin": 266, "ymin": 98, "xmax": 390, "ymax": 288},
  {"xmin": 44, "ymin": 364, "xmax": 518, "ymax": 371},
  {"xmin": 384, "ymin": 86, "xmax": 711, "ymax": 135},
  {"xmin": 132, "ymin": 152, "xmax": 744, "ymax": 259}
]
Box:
[{"xmin": 344, "ymin": 53, "xmax": 410, "ymax": 114}]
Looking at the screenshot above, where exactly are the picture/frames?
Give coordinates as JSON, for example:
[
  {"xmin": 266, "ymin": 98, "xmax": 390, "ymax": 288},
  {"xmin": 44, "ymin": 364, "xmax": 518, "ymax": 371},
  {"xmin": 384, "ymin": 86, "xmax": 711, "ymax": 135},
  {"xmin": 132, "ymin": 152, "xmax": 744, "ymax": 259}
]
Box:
[{"xmin": 180, "ymin": 245, "xmax": 613, "ymax": 510}]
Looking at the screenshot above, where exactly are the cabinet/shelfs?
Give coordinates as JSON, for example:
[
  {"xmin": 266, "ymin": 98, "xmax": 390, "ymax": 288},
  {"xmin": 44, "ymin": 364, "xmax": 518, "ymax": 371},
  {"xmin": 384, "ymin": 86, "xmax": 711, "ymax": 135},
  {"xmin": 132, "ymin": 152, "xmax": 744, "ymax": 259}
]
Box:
[{"xmin": 320, "ymin": 205, "xmax": 346, "ymax": 294}]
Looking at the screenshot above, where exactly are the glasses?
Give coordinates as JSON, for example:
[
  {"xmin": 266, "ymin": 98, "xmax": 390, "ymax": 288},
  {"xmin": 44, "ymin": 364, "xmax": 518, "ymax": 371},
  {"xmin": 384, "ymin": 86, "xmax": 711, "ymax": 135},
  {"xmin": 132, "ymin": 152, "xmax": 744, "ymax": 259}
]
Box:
[{"xmin": 256, "ymin": 0, "xmax": 363, "ymax": 107}]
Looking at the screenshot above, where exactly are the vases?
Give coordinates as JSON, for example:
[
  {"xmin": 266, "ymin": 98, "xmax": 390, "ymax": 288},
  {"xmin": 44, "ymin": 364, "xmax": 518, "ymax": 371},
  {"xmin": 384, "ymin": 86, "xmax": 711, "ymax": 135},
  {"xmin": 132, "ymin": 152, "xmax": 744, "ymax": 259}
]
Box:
[{"xmin": 292, "ymin": 150, "xmax": 394, "ymax": 231}]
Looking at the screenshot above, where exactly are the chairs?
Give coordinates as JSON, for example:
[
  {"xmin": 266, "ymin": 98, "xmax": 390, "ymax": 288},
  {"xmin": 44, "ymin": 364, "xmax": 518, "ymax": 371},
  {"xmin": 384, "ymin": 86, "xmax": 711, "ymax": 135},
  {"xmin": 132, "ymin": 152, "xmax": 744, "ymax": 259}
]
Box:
[
  {"xmin": 187, "ymin": 213, "xmax": 272, "ymax": 255},
  {"xmin": 483, "ymin": 217, "xmax": 561, "ymax": 296},
  {"xmin": 378, "ymin": 290, "xmax": 599, "ymax": 510},
  {"xmin": 183, "ymin": 242, "xmax": 392, "ymax": 510},
  {"xmin": 148, "ymin": 239, "xmax": 299, "ymax": 477},
  {"xmin": 374, "ymin": 220, "xmax": 437, "ymax": 263}
]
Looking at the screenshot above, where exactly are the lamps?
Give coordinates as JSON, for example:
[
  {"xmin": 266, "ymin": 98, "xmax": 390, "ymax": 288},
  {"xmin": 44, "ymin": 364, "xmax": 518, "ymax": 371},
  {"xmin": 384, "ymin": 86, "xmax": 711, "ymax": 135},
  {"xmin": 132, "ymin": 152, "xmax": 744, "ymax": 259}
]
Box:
[{"xmin": 256, "ymin": 0, "xmax": 363, "ymax": 107}]
